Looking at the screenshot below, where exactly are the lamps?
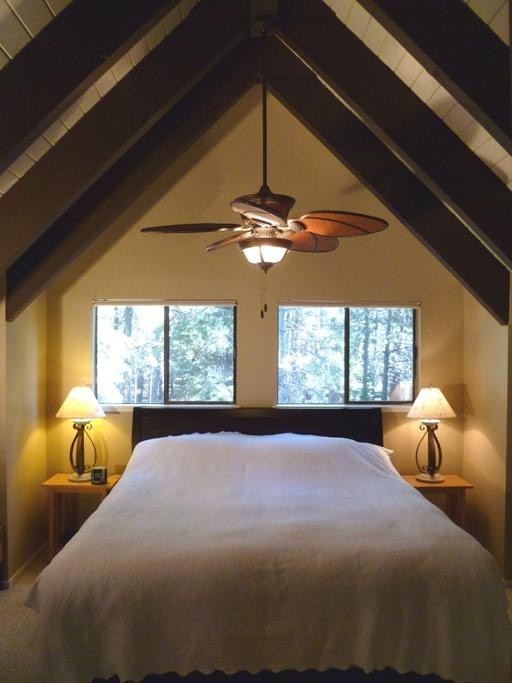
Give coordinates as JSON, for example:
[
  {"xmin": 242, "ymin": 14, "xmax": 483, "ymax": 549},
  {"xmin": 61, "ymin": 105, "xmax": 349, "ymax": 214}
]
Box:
[
  {"xmin": 406, "ymin": 385, "xmax": 457, "ymax": 483},
  {"xmin": 237, "ymin": 237, "xmax": 293, "ymax": 319},
  {"xmin": 56, "ymin": 386, "xmax": 105, "ymax": 483}
]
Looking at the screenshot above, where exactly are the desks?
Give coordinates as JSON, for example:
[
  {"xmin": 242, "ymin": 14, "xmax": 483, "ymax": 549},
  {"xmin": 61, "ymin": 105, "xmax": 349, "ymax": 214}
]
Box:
[
  {"xmin": 41, "ymin": 473, "xmax": 120, "ymax": 562},
  {"xmin": 403, "ymin": 475, "xmax": 473, "ymax": 527}
]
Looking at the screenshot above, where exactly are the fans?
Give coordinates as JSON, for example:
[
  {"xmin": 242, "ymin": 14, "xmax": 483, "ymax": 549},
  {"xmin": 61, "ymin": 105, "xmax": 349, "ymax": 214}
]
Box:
[{"xmin": 141, "ymin": 16, "xmax": 388, "ymax": 253}]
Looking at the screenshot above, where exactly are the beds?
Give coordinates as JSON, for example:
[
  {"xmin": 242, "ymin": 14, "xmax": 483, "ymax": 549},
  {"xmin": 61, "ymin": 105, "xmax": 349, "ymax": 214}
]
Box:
[{"xmin": 24, "ymin": 406, "xmax": 512, "ymax": 683}]
